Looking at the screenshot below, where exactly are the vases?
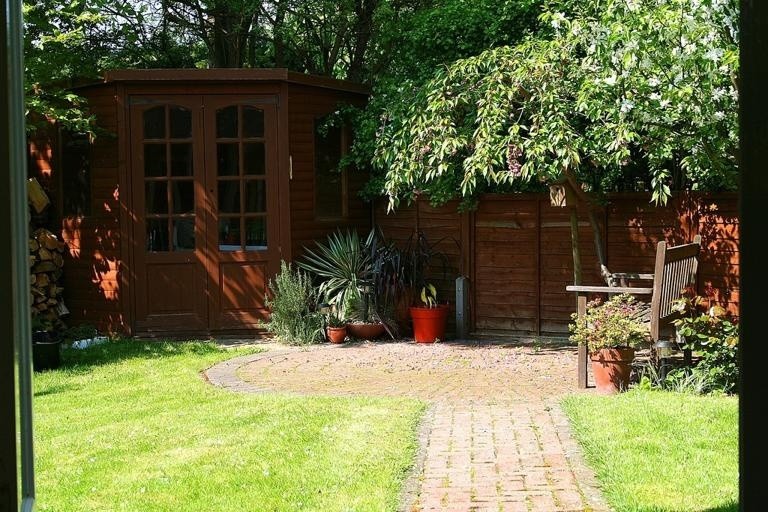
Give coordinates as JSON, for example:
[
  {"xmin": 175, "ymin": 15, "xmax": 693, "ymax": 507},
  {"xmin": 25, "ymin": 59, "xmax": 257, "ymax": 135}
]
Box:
[{"xmin": 29, "ymin": 330, "xmax": 62, "ymax": 371}]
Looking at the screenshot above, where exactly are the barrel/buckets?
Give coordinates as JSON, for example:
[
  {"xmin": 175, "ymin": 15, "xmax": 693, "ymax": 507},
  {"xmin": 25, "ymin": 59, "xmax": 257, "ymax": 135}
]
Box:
[
  {"xmin": 409, "ymin": 302, "xmax": 450, "ymax": 343},
  {"xmin": 588, "ymin": 350, "xmax": 634, "ymax": 398}
]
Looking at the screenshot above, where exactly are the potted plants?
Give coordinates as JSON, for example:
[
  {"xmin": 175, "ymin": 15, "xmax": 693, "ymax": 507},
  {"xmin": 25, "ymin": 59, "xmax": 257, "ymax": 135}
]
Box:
[
  {"xmin": 564, "ymin": 293, "xmax": 655, "ymax": 397},
  {"xmin": 296, "ymin": 230, "xmax": 451, "ymax": 346}
]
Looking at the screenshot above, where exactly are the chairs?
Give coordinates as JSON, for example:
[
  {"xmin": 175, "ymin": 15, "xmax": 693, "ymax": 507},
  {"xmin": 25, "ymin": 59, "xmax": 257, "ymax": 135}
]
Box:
[{"xmin": 565, "ymin": 235, "xmax": 705, "ymax": 395}]
